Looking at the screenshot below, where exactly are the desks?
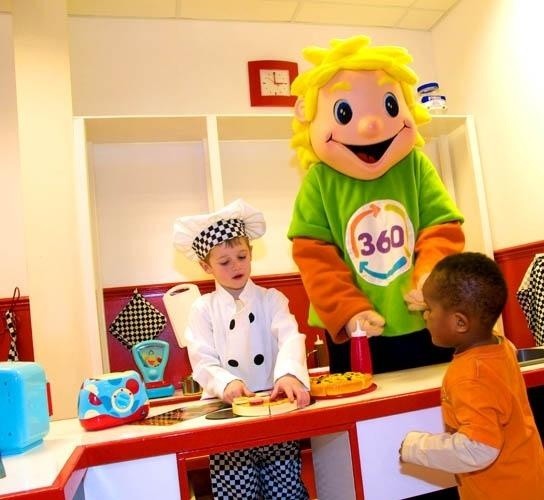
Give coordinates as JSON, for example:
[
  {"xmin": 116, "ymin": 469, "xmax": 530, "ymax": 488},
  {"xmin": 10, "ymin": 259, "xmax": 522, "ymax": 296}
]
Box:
[{"xmin": 0, "ymin": 344, "xmax": 544, "ymax": 500}]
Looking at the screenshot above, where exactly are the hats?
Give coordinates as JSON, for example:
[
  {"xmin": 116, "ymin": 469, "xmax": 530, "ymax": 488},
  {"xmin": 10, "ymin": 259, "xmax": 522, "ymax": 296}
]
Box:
[{"xmin": 170, "ymin": 197, "xmax": 266, "ymax": 262}]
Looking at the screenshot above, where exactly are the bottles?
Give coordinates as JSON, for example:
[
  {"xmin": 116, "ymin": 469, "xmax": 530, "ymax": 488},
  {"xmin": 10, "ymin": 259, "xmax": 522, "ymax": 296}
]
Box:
[
  {"xmin": 314, "ymin": 332, "xmax": 328, "ymax": 367},
  {"xmin": 418, "ymin": 82, "xmax": 447, "ymax": 114},
  {"xmin": 350, "ymin": 320, "xmax": 373, "ymax": 374}
]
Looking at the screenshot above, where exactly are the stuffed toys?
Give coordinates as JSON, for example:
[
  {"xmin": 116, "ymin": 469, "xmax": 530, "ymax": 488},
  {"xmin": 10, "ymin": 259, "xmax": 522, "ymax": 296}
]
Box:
[{"xmin": 281, "ymin": 32, "xmax": 466, "ymax": 376}]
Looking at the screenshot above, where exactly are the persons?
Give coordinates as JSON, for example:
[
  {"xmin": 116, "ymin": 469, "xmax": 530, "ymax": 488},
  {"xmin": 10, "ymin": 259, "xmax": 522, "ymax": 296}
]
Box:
[
  {"xmin": 396, "ymin": 252, "xmax": 544, "ymax": 499},
  {"xmin": 171, "ymin": 196, "xmax": 312, "ymax": 500}
]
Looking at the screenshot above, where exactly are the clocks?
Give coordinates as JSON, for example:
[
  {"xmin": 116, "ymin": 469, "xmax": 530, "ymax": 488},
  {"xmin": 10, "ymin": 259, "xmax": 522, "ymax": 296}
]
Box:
[{"xmin": 248, "ymin": 60, "xmax": 298, "ymax": 107}]
